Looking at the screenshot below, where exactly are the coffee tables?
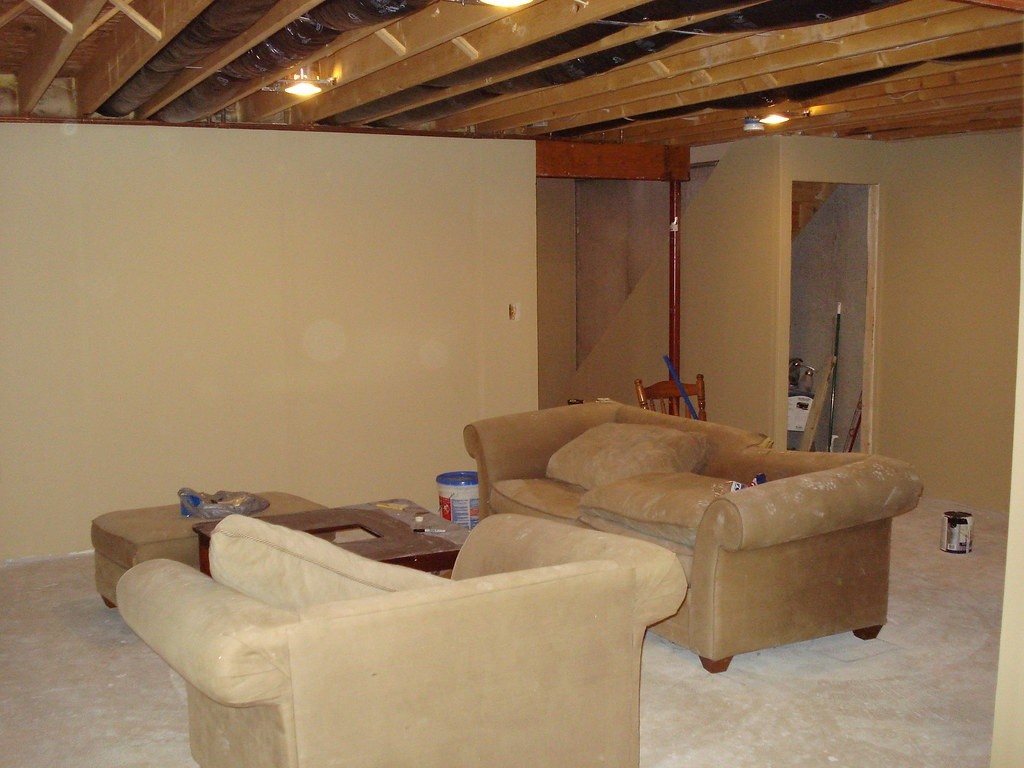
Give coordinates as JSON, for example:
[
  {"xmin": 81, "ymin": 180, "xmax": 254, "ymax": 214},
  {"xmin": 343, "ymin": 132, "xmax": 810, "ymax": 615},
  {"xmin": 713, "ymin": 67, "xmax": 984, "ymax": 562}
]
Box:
[
  {"xmin": 192, "ymin": 510, "xmax": 461, "ymax": 576},
  {"xmin": 192, "ymin": 498, "xmax": 471, "ymax": 577}
]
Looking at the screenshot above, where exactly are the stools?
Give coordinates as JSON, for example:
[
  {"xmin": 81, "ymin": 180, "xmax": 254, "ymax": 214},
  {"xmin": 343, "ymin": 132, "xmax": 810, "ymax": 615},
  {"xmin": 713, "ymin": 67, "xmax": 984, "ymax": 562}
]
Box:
[{"xmin": 91, "ymin": 491, "xmax": 326, "ymax": 609}]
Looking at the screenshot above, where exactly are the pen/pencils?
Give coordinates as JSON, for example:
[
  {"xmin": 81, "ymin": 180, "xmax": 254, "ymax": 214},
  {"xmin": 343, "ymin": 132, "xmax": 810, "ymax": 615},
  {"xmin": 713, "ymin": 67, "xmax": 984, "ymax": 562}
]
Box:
[{"xmin": 413, "ymin": 528, "xmax": 446, "ymax": 533}]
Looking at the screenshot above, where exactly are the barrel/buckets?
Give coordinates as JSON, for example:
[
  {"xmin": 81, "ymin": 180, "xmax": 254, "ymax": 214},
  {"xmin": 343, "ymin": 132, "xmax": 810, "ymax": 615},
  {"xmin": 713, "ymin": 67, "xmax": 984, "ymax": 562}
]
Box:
[
  {"xmin": 435, "ymin": 472, "xmax": 480, "ymax": 529},
  {"xmin": 940, "ymin": 511, "xmax": 973, "ymax": 553}
]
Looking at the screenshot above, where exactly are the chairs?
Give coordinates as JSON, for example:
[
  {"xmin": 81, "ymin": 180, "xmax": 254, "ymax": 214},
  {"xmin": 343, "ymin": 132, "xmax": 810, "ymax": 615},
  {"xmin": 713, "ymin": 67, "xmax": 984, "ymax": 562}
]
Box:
[{"xmin": 634, "ymin": 374, "xmax": 706, "ymax": 420}]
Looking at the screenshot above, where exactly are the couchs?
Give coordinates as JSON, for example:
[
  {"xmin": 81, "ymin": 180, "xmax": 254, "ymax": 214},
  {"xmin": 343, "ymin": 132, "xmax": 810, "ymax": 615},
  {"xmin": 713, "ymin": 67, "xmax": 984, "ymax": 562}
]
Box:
[
  {"xmin": 463, "ymin": 400, "xmax": 923, "ymax": 674},
  {"xmin": 117, "ymin": 514, "xmax": 688, "ymax": 768}
]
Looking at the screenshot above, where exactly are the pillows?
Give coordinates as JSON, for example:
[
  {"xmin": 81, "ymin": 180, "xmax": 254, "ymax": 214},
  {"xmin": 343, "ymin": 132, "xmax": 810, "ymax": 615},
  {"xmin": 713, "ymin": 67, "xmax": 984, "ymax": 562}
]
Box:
[
  {"xmin": 212, "ymin": 515, "xmax": 456, "ymax": 608},
  {"xmin": 578, "ymin": 472, "xmax": 747, "ymax": 548},
  {"xmin": 544, "ymin": 422, "xmax": 708, "ymax": 492}
]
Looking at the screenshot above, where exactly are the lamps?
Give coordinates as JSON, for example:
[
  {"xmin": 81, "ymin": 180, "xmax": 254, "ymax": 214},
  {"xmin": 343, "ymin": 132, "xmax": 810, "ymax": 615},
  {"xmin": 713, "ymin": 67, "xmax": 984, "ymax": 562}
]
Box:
[
  {"xmin": 740, "ymin": 98, "xmax": 811, "ymax": 124},
  {"xmin": 262, "ymin": 69, "xmax": 336, "ymax": 97}
]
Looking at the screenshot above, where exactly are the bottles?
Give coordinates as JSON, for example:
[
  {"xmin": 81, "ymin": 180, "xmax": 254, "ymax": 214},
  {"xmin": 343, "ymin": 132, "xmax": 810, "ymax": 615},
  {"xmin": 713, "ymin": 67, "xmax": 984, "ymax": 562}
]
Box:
[
  {"xmin": 799, "ymin": 366, "xmax": 816, "ymax": 392},
  {"xmin": 789, "ymin": 357, "xmax": 803, "ymax": 386},
  {"xmin": 711, "ymin": 481, "xmax": 746, "ymax": 495}
]
